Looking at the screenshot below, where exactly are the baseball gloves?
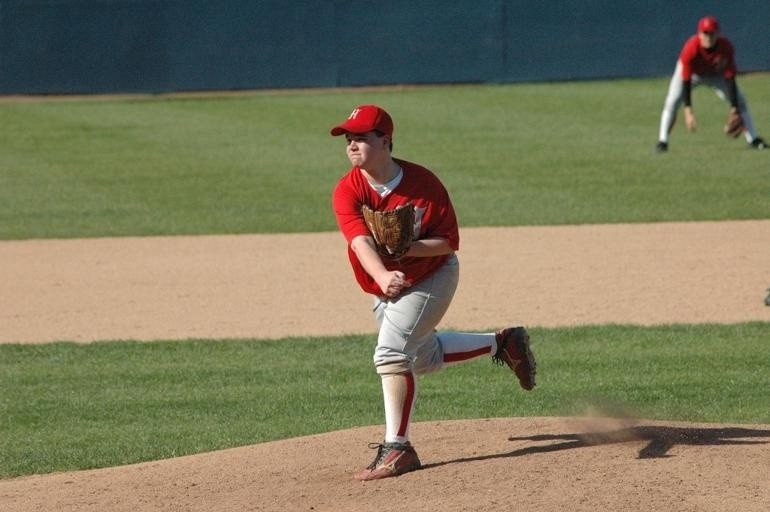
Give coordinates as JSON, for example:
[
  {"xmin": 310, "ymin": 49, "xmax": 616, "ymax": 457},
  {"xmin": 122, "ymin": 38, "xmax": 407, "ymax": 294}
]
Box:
[
  {"xmin": 361, "ymin": 203, "xmax": 414, "ymax": 256},
  {"xmin": 724, "ymin": 111, "xmax": 744, "ymax": 137}
]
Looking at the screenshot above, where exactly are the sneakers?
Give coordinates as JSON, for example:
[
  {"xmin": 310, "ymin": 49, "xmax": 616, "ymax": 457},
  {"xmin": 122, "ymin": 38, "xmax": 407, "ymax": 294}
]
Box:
[
  {"xmin": 657, "ymin": 142, "xmax": 667, "ymax": 152},
  {"xmin": 492, "ymin": 327, "xmax": 537, "ymax": 391},
  {"xmin": 355, "ymin": 442, "xmax": 421, "ymax": 481},
  {"xmin": 749, "ymin": 137, "xmax": 767, "ymax": 150}
]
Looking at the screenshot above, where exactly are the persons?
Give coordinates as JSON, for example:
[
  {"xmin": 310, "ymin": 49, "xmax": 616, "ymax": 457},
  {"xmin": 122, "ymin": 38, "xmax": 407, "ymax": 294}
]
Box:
[
  {"xmin": 656, "ymin": 14, "xmax": 769, "ymax": 151},
  {"xmin": 327, "ymin": 103, "xmax": 536, "ymax": 482}
]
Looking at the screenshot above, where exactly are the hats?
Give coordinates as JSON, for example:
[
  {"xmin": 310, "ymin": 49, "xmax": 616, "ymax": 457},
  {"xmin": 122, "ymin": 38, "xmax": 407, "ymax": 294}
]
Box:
[
  {"xmin": 331, "ymin": 105, "xmax": 394, "ymax": 137},
  {"xmin": 699, "ymin": 17, "xmax": 719, "ymax": 33}
]
